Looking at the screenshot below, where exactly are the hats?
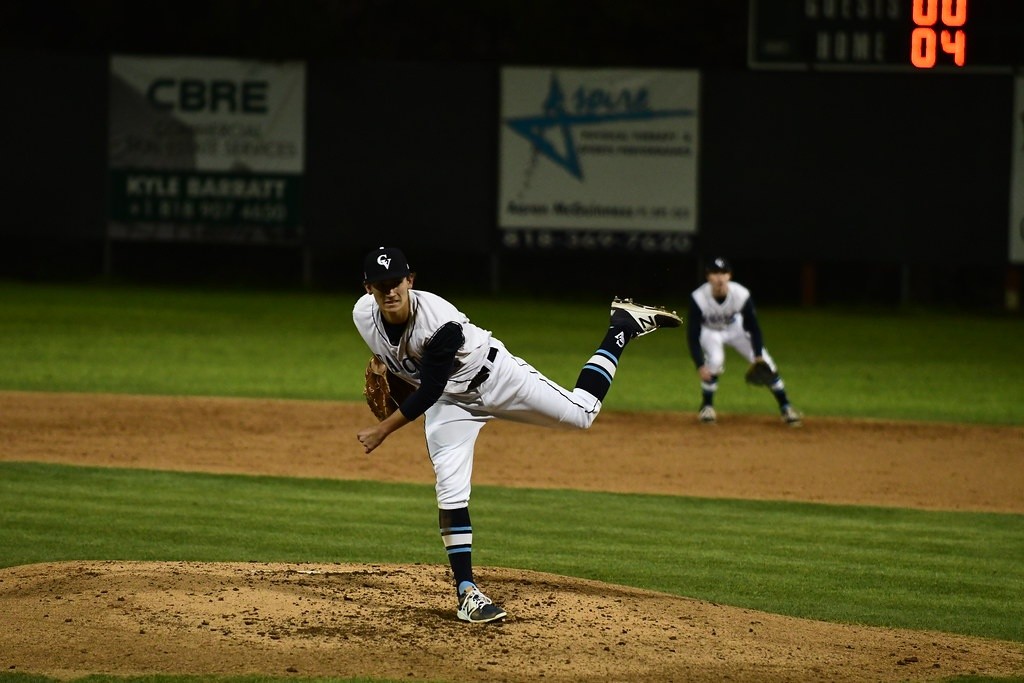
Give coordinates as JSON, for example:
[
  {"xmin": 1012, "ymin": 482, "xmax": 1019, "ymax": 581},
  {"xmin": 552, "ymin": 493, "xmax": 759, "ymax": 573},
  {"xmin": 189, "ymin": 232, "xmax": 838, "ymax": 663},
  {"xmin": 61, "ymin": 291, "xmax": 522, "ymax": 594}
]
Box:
[
  {"xmin": 364, "ymin": 245, "xmax": 413, "ymax": 285},
  {"xmin": 706, "ymin": 256, "xmax": 732, "ymax": 273}
]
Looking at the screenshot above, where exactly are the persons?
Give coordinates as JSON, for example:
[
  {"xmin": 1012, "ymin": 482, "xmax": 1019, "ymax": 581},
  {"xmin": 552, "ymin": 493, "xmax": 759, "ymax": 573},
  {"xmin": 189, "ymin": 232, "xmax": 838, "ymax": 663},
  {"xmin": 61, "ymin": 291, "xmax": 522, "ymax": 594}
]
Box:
[
  {"xmin": 687, "ymin": 258, "xmax": 799, "ymax": 423},
  {"xmin": 353, "ymin": 247, "xmax": 684, "ymax": 624}
]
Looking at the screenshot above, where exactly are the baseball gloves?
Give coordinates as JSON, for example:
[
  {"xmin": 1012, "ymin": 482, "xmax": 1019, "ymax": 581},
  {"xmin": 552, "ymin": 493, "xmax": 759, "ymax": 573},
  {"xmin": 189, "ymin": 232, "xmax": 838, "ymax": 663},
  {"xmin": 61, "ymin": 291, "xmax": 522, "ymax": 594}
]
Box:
[
  {"xmin": 363, "ymin": 354, "xmax": 398, "ymax": 423},
  {"xmin": 744, "ymin": 358, "xmax": 781, "ymax": 388}
]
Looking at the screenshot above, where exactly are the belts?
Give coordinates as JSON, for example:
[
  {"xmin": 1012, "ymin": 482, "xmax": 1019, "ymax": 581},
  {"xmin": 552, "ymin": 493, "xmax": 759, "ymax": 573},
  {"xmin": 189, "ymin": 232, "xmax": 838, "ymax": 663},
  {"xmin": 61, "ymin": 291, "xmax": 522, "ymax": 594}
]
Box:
[{"xmin": 467, "ymin": 347, "xmax": 498, "ymax": 390}]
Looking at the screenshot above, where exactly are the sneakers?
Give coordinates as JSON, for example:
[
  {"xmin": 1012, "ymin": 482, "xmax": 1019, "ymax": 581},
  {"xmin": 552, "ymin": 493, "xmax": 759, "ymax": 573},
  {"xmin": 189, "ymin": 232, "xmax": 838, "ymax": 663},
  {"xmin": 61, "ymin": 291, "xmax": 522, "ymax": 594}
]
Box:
[
  {"xmin": 457, "ymin": 586, "xmax": 507, "ymax": 622},
  {"xmin": 781, "ymin": 409, "xmax": 803, "ymax": 427},
  {"xmin": 609, "ymin": 296, "xmax": 684, "ymax": 339},
  {"xmin": 699, "ymin": 405, "xmax": 716, "ymax": 424}
]
